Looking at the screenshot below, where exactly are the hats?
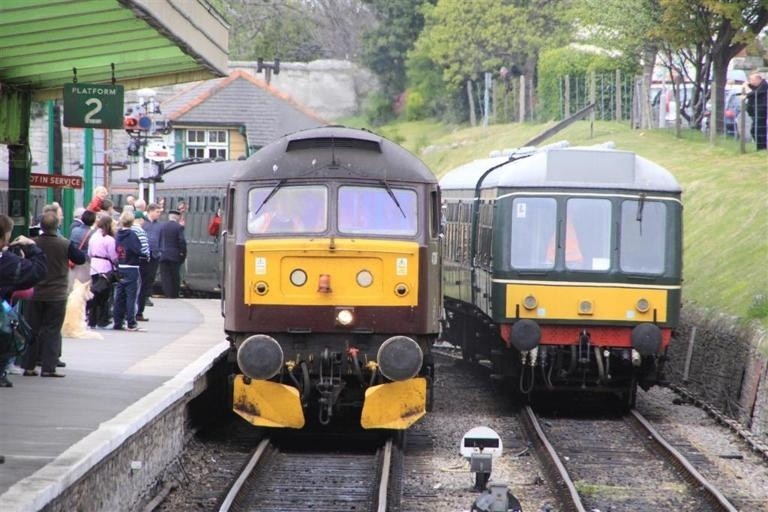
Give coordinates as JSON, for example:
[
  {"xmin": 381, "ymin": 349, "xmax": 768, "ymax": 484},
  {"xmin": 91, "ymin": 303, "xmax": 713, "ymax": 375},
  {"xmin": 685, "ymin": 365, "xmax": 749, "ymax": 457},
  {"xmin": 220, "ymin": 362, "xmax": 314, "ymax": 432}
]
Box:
[
  {"xmin": 97, "ymin": 217, "xmax": 111, "ymax": 227},
  {"xmin": 168, "ymin": 211, "xmax": 180, "ymax": 215}
]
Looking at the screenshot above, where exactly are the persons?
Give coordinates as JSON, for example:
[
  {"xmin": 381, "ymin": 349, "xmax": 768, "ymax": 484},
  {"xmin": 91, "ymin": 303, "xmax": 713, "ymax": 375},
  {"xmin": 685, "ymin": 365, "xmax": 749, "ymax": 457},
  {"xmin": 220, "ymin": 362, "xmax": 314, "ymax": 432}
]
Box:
[
  {"xmin": 0, "ymin": 212, "xmax": 50, "ymax": 467},
  {"xmin": 743, "ymin": 71, "xmax": 767, "ymax": 150},
  {"xmin": 251, "ymin": 193, "xmax": 307, "ymax": 231},
  {"xmin": 0, "ymin": 183, "xmax": 189, "ymax": 388},
  {"xmin": 703, "ymin": 80, "xmax": 741, "ymax": 134},
  {"xmin": 207, "ymin": 206, "xmax": 222, "ymax": 236}
]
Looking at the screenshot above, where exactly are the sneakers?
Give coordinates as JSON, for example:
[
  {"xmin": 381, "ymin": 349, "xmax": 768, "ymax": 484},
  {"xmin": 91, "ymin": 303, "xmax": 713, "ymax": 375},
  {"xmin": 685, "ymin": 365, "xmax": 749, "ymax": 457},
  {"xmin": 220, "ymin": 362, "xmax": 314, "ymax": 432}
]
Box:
[
  {"xmin": 0, "ymin": 360, "xmax": 66, "ymax": 387},
  {"xmin": 86, "ymin": 298, "xmax": 154, "ymax": 333}
]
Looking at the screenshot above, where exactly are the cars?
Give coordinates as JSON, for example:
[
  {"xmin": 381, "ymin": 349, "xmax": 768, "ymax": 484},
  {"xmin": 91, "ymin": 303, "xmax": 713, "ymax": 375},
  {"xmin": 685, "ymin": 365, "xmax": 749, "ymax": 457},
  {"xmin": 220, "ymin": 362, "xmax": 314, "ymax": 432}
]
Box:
[{"xmin": 640, "ymin": 78, "xmax": 754, "ymax": 142}]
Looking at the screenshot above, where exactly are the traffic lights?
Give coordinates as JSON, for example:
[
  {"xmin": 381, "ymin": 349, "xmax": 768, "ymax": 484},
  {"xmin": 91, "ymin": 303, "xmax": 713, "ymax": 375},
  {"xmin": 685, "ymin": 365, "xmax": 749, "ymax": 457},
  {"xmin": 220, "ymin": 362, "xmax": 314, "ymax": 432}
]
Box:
[{"xmin": 121, "ymin": 115, "xmax": 155, "ymax": 132}]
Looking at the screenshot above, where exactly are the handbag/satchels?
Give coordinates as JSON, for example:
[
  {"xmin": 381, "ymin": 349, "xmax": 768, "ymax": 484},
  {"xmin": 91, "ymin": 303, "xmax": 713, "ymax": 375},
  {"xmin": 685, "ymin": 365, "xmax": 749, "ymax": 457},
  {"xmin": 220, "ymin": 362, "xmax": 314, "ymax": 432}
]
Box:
[{"xmin": 0, "ymin": 298, "xmax": 31, "ymax": 355}]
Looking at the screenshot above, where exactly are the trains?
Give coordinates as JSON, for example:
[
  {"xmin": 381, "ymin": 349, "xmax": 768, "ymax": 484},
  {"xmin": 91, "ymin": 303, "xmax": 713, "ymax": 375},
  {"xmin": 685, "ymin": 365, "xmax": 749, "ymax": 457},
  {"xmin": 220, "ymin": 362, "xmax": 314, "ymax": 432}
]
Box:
[
  {"xmin": 426, "ymin": 137, "xmax": 685, "ymax": 416},
  {"xmin": 0, "ymin": 126, "xmax": 443, "ymax": 436}
]
